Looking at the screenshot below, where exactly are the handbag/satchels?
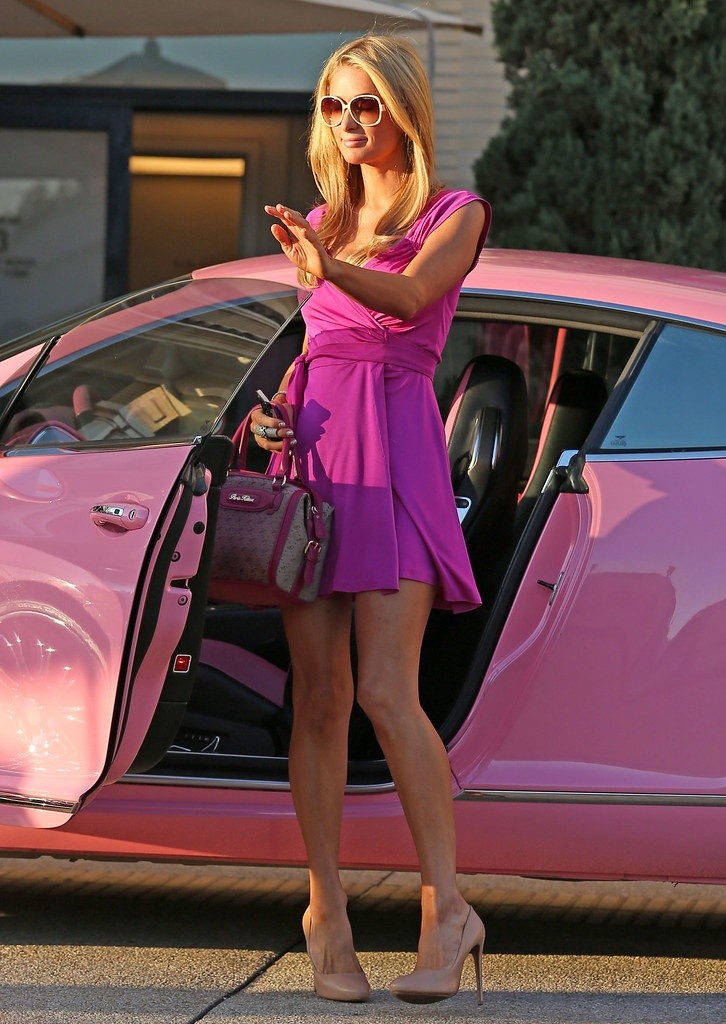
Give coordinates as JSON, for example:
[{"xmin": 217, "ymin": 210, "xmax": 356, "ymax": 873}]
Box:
[{"xmin": 207, "ymin": 401, "xmax": 335, "ymax": 606}]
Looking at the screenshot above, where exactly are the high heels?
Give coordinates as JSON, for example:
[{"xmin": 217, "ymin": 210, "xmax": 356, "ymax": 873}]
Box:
[
  {"xmin": 302, "ymin": 905, "xmax": 370, "ymax": 1000},
  {"xmin": 390, "ymin": 905, "xmax": 485, "ymax": 1006}
]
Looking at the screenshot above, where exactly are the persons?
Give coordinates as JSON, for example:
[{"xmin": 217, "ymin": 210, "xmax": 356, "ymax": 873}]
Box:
[{"xmin": 242, "ymin": 39, "xmax": 487, "ymax": 1006}]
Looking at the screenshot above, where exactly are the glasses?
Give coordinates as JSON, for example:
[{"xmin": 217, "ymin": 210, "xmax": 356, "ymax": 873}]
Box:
[{"xmin": 319, "ymin": 94, "xmax": 407, "ymax": 127}]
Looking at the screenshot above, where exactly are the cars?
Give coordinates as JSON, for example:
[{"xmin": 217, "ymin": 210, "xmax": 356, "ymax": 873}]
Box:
[{"xmin": 1, "ymin": 241, "xmax": 726, "ymax": 885}]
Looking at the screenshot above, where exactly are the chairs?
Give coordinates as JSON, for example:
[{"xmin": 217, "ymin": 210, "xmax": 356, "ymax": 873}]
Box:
[
  {"xmin": 179, "ymin": 369, "xmax": 611, "ymax": 760},
  {"xmin": 444, "ymin": 355, "xmax": 527, "ymax": 552}
]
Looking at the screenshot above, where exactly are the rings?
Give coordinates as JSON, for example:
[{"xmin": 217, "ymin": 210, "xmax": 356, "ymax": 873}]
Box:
[{"xmin": 254, "ymin": 424, "xmax": 277, "ymax": 439}]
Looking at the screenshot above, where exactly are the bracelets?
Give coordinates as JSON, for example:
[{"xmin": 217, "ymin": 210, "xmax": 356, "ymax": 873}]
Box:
[{"xmin": 272, "ymin": 391, "xmax": 289, "ymax": 401}]
